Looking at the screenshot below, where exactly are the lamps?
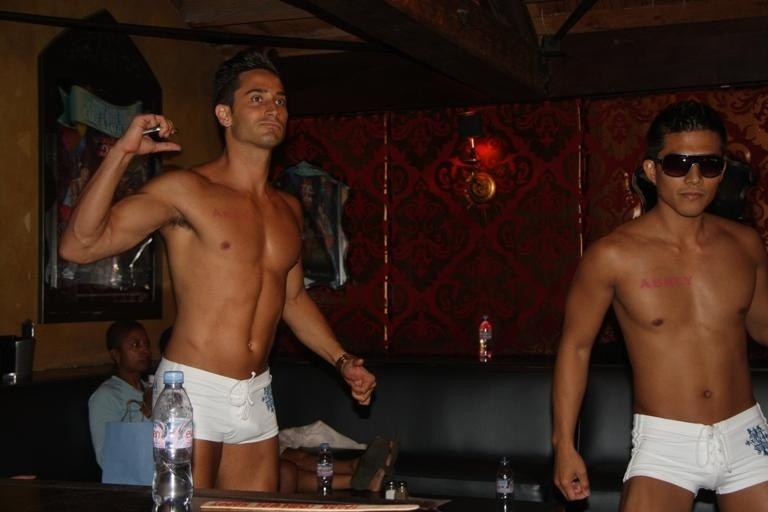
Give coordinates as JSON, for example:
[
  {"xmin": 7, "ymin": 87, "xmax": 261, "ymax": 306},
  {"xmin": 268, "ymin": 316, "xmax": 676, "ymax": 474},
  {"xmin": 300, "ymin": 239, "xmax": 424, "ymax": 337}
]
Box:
[{"xmin": 464, "ymin": 137, "xmax": 496, "ymax": 218}]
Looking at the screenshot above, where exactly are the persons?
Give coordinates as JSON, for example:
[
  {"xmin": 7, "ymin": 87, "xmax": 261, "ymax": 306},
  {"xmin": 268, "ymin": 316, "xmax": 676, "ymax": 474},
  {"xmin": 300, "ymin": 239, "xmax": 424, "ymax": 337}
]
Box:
[
  {"xmin": 57, "ymin": 48, "xmax": 396, "ymax": 493},
  {"xmin": 550, "ymin": 101, "xmax": 768, "ymax": 512}
]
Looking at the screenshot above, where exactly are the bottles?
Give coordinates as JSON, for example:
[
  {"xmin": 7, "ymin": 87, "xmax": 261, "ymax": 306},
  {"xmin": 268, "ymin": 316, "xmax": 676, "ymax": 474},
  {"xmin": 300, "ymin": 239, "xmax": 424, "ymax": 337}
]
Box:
[
  {"xmin": 476, "ymin": 313, "xmax": 493, "ymax": 363},
  {"xmin": 494, "ymin": 457, "xmax": 517, "ymax": 512},
  {"xmin": 150, "ymin": 371, "xmax": 195, "ymax": 511},
  {"xmin": 315, "ymin": 441, "xmax": 336, "ymax": 499},
  {"xmin": 384, "ymin": 480, "xmax": 409, "ymax": 502}
]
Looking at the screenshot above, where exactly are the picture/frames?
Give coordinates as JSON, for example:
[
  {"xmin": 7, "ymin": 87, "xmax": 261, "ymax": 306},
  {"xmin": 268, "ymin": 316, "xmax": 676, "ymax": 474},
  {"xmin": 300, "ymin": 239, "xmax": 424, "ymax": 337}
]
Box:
[{"xmin": 38, "ymin": 7, "xmax": 163, "ymax": 327}]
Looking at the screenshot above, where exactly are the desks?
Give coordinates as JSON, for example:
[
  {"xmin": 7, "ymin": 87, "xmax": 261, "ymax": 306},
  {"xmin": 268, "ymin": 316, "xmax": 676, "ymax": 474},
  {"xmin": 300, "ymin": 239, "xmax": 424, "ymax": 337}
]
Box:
[{"xmin": 0, "ymin": 478, "xmax": 452, "ymax": 512}]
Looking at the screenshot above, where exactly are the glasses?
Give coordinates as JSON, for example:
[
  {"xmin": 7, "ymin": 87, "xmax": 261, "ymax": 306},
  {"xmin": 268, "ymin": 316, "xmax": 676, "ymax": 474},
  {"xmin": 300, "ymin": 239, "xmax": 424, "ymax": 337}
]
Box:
[{"xmin": 656, "ymin": 153, "xmax": 728, "ymax": 178}]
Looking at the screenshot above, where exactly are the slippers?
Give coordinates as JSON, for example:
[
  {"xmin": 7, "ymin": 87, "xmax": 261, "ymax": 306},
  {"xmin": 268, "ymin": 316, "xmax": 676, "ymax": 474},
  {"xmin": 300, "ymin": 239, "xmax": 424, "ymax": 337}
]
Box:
[{"xmin": 349, "ymin": 435, "xmax": 399, "ymax": 492}]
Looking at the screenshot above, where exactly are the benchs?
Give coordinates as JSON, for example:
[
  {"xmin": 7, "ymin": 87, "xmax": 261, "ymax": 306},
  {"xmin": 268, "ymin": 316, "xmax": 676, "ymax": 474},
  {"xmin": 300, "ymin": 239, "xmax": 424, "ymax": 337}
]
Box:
[{"xmin": 0, "ymin": 355, "xmax": 720, "ymax": 511}]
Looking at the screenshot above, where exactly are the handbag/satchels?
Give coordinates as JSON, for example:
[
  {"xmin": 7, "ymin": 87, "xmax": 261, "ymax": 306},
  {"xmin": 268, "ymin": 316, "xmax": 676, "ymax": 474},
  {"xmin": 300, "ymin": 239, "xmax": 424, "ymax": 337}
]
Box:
[{"xmin": 103, "ymin": 400, "xmax": 155, "ymax": 487}]
